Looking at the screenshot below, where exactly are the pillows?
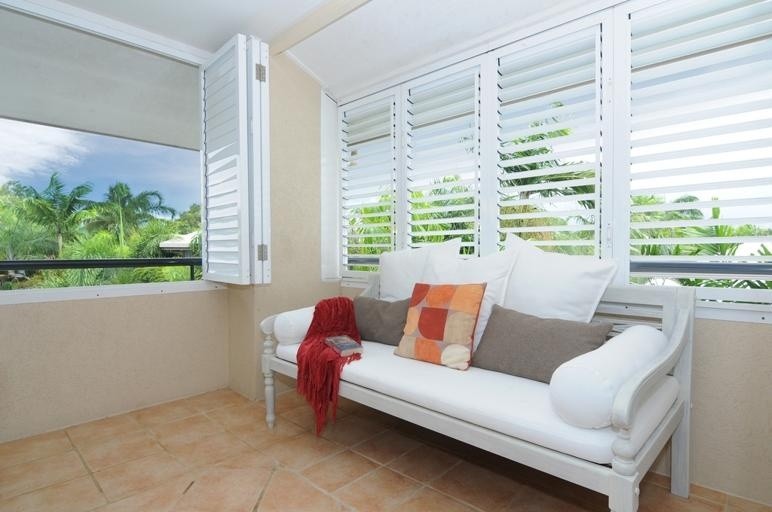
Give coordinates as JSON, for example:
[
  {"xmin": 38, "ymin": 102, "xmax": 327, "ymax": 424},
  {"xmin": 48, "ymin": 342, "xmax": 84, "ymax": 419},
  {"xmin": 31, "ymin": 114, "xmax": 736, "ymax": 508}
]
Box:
[
  {"xmin": 378, "ymin": 236, "xmax": 464, "ymax": 302},
  {"xmin": 469, "ymin": 303, "xmax": 614, "ymax": 386},
  {"xmin": 393, "ymin": 281, "xmax": 488, "ymax": 371},
  {"xmin": 351, "ymin": 296, "xmax": 410, "ymax": 346},
  {"xmin": 418, "ymin": 246, "xmax": 522, "ymax": 360},
  {"xmin": 505, "ymin": 231, "xmax": 619, "ymax": 323}
]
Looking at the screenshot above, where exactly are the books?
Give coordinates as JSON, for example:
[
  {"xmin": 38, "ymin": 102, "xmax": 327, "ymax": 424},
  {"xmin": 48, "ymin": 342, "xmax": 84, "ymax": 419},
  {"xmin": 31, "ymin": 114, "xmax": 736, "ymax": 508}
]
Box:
[{"xmin": 324, "ymin": 334, "xmax": 364, "ymax": 358}]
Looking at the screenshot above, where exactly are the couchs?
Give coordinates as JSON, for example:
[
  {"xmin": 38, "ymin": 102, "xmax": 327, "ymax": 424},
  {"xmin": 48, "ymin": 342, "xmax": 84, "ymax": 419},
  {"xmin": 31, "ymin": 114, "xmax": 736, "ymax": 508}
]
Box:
[{"xmin": 261, "ymin": 274, "xmax": 695, "ymax": 512}]
